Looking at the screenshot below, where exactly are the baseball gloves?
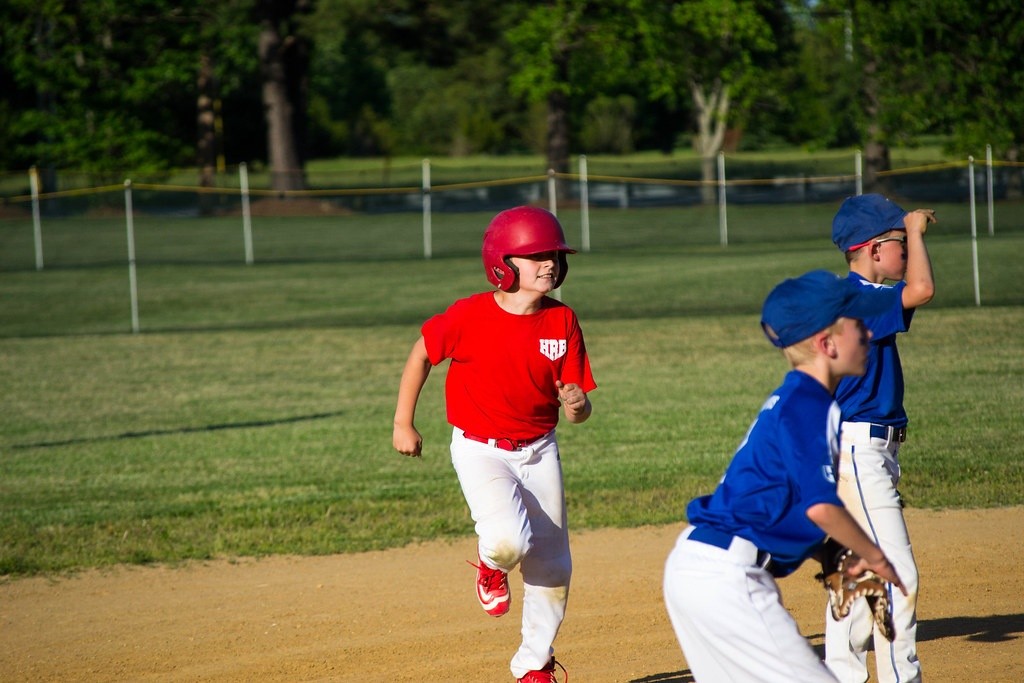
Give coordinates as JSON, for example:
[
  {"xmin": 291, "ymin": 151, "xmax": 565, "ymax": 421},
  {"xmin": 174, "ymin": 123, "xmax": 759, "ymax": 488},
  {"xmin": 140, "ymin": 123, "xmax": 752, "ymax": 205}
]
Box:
[{"xmin": 814, "ymin": 533, "xmax": 898, "ymax": 643}]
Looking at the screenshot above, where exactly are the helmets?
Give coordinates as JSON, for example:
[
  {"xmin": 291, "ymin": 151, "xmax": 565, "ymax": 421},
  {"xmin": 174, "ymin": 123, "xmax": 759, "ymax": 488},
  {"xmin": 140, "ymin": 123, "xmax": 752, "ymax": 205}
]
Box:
[{"xmin": 482, "ymin": 206, "xmax": 578, "ymax": 290}]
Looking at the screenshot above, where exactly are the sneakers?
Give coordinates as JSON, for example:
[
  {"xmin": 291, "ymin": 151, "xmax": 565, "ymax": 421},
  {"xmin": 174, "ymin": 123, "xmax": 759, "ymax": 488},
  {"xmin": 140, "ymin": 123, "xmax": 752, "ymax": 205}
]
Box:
[
  {"xmin": 466, "ymin": 544, "xmax": 511, "ymax": 617},
  {"xmin": 517, "ymin": 656, "xmax": 568, "ymax": 683}
]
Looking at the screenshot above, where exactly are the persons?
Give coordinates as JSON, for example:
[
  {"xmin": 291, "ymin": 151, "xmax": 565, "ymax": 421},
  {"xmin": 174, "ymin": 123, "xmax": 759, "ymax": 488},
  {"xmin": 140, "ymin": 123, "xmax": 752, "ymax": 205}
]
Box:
[
  {"xmin": 661, "ymin": 269, "xmax": 919, "ymax": 683},
  {"xmin": 823, "ymin": 192, "xmax": 937, "ymax": 683},
  {"xmin": 392, "ymin": 204, "xmax": 598, "ymax": 683}
]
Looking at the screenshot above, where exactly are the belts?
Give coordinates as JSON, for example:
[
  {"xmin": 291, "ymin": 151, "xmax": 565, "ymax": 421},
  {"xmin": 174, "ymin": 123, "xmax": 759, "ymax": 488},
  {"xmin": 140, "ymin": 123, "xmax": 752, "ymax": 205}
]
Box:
[
  {"xmin": 463, "ymin": 432, "xmax": 545, "ymax": 451},
  {"xmin": 839, "ymin": 422, "xmax": 906, "ymax": 442},
  {"xmin": 688, "ymin": 526, "xmax": 783, "ymax": 578}
]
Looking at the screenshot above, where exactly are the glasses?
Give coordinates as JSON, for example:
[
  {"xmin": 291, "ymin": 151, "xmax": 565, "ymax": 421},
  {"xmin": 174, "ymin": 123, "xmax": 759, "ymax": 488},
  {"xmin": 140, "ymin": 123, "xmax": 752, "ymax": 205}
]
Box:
[{"xmin": 850, "ymin": 234, "xmax": 906, "ymax": 252}]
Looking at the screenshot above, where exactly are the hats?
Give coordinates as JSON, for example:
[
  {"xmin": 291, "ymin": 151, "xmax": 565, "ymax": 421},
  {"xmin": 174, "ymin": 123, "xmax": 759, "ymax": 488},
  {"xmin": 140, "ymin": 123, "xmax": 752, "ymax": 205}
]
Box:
[
  {"xmin": 830, "ymin": 193, "xmax": 909, "ymax": 252},
  {"xmin": 760, "ymin": 270, "xmax": 856, "ymax": 348}
]
